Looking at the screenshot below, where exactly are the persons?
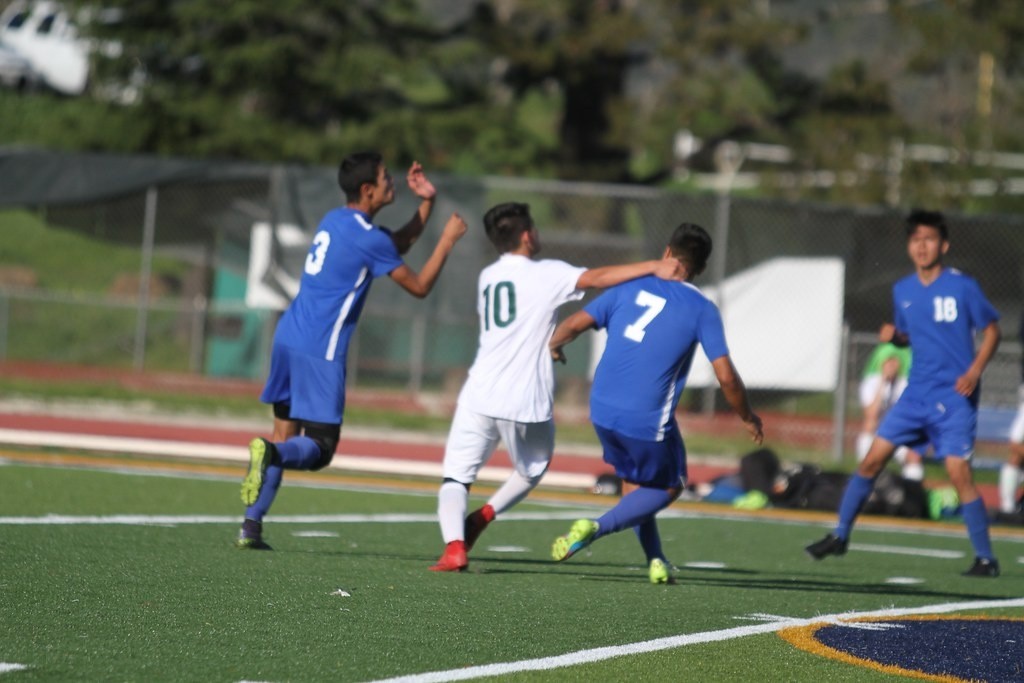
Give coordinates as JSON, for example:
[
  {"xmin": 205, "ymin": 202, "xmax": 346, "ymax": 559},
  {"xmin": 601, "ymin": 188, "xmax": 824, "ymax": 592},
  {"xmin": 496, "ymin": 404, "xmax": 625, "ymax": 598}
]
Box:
[
  {"xmin": 855, "ymin": 326, "xmax": 924, "ymax": 482},
  {"xmin": 803, "ymin": 211, "xmax": 1002, "ymax": 579},
  {"xmin": 997, "ymin": 403, "xmax": 1024, "ymax": 513},
  {"xmin": 238, "ymin": 152, "xmax": 468, "ymax": 548},
  {"xmin": 549, "ymin": 223, "xmax": 764, "ymax": 581},
  {"xmin": 427, "ymin": 202, "xmax": 688, "ymax": 572}
]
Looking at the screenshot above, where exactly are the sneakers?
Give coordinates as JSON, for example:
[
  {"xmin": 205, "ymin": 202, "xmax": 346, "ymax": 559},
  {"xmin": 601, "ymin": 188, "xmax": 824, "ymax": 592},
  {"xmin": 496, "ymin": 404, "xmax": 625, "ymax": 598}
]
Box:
[
  {"xmin": 806, "ymin": 535, "xmax": 848, "ymax": 560},
  {"xmin": 461, "ymin": 507, "xmax": 496, "ymax": 552},
  {"xmin": 237, "ymin": 527, "xmax": 270, "ymax": 550},
  {"xmin": 240, "ymin": 435, "xmax": 272, "ymax": 506},
  {"xmin": 429, "ymin": 542, "xmax": 467, "ymax": 571},
  {"xmin": 552, "ymin": 519, "xmax": 596, "ymax": 560},
  {"xmin": 962, "ymin": 557, "xmax": 999, "ymax": 576},
  {"xmin": 649, "ymin": 558, "xmax": 677, "ymax": 583}
]
